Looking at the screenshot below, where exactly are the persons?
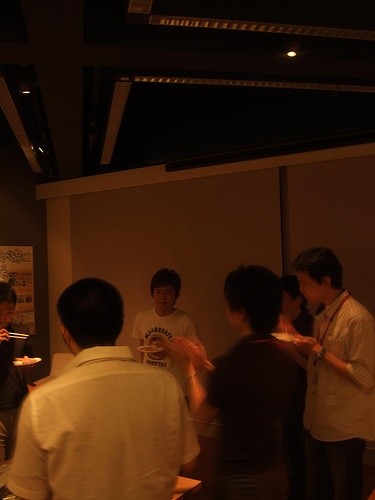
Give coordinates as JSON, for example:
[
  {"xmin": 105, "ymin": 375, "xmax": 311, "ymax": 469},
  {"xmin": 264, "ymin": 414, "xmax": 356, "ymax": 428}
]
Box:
[
  {"xmin": 273, "ymin": 245, "xmax": 375, "ymax": 500},
  {"xmin": 166, "ymin": 265, "xmax": 310, "ymax": 500},
  {"xmin": 276, "ymin": 274, "xmax": 316, "ymax": 340},
  {"xmin": 0, "ymin": 282, "xmax": 36, "ymax": 500},
  {"xmin": 131, "ymin": 266, "xmax": 198, "ymax": 413},
  {"xmin": 5, "ymin": 277, "xmax": 202, "ymax": 499}
]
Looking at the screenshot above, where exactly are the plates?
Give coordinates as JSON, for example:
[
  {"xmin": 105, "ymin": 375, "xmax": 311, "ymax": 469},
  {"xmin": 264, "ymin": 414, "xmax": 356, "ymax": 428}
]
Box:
[
  {"xmin": 138, "ymin": 345, "xmax": 163, "ymax": 353},
  {"xmin": 13, "ymin": 357, "xmax": 42, "ymax": 366},
  {"xmin": 269, "ymin": 333, "xmax": 309, "ymax": 343}
]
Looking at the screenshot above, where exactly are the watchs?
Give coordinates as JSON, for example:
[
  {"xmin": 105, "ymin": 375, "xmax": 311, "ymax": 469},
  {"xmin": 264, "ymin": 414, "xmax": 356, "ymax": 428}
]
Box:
[{"xmin": 314, "ymin": 348, "xmax": 326, "ymax": 362}]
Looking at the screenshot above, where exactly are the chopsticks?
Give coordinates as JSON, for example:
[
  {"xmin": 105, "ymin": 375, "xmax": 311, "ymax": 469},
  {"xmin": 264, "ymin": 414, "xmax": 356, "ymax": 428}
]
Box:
[{"xmin": 8, "ymin": 332, "xmax": 29, "ymax": 340}]
